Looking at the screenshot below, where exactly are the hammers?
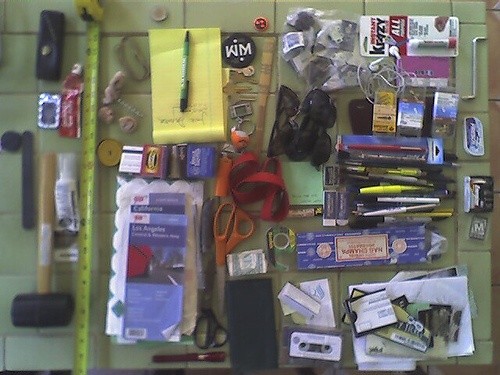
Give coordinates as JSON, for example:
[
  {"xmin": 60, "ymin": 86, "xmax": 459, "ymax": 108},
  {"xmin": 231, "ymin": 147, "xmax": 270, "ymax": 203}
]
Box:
[{"xmin": 10, "ymin": 153, "xmax": 74, "ymax": 327}]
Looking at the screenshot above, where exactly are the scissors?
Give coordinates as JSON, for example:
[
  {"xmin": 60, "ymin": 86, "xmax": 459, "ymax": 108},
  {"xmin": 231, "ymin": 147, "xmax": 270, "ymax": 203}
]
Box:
[
  {"xmin": 214, "ymin": 201, "xmax": 256, "ymax": 317},
  {"xmin": 193, "ymin": 198, "xmax": 230, "ymax": 349}
]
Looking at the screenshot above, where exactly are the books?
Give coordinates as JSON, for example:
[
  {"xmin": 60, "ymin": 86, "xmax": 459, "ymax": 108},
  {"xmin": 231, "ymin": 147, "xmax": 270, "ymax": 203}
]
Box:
[{"xmin": 148, "ymin": 27, "xmax": 231, "ymax": 145}]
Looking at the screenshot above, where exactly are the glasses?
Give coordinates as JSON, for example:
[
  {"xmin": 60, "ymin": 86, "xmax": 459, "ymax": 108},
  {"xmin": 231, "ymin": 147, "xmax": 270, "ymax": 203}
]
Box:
[{"xmin": 266, "ymin": 84, "xmax": 337, "ymax": 172}]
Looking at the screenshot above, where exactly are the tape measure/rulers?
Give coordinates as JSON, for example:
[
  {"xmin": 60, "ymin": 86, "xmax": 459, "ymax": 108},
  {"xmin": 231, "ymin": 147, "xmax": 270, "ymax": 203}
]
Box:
[{"xmin": 73, "ymin": 0, "xmax": 105, "ymax": 375}]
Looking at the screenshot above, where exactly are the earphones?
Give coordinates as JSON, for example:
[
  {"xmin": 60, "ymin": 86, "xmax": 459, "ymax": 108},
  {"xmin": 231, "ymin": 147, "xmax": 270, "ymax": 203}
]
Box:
[
  {"xmin": 369, "ymin": 58, "xmax": 384, "ymax": 72},
  {"xmin": 389, "ymin": 45, "xmax": 402, "ymax": 61}
]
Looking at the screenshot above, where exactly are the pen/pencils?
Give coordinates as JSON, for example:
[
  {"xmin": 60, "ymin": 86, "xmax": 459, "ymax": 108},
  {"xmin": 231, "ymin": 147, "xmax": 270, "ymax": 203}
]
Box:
[
  {"xmin": 152, "ymin": 352, "xmax": 225, "ymax": 363},
  {"xmin": 21, "ymin": 131, "xmax": 34, "ymax": 229},
  {"xmin": 179, "ymin": 30, "xmax": 190, "ymax": 112},
  {"xmin": 340, "ymin": 144, "xmax": 462, "ymax": 185},
  {"xmin": 350, "ymin": 186, "xmax": 457, "ymax": 226}
]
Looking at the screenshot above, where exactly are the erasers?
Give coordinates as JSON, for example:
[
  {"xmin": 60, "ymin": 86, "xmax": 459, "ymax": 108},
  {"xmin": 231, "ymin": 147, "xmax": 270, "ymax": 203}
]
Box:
[
  {"xmin": 323, "ymin": 189, "xmax": 337, "ymax": 226},
  {"xmin": 337, "ymin": 192, "xmax": 348, "ymax": 226},
  {"xmin": 324, "ymin": 165, "xmax": 340, "ymax": 188}
]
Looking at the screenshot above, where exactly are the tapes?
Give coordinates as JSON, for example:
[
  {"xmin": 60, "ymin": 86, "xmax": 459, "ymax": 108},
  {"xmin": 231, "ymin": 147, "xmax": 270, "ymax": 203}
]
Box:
[{"xmin": 264, "ymin": 223, "xmax": 296, "ymax": 272}]
